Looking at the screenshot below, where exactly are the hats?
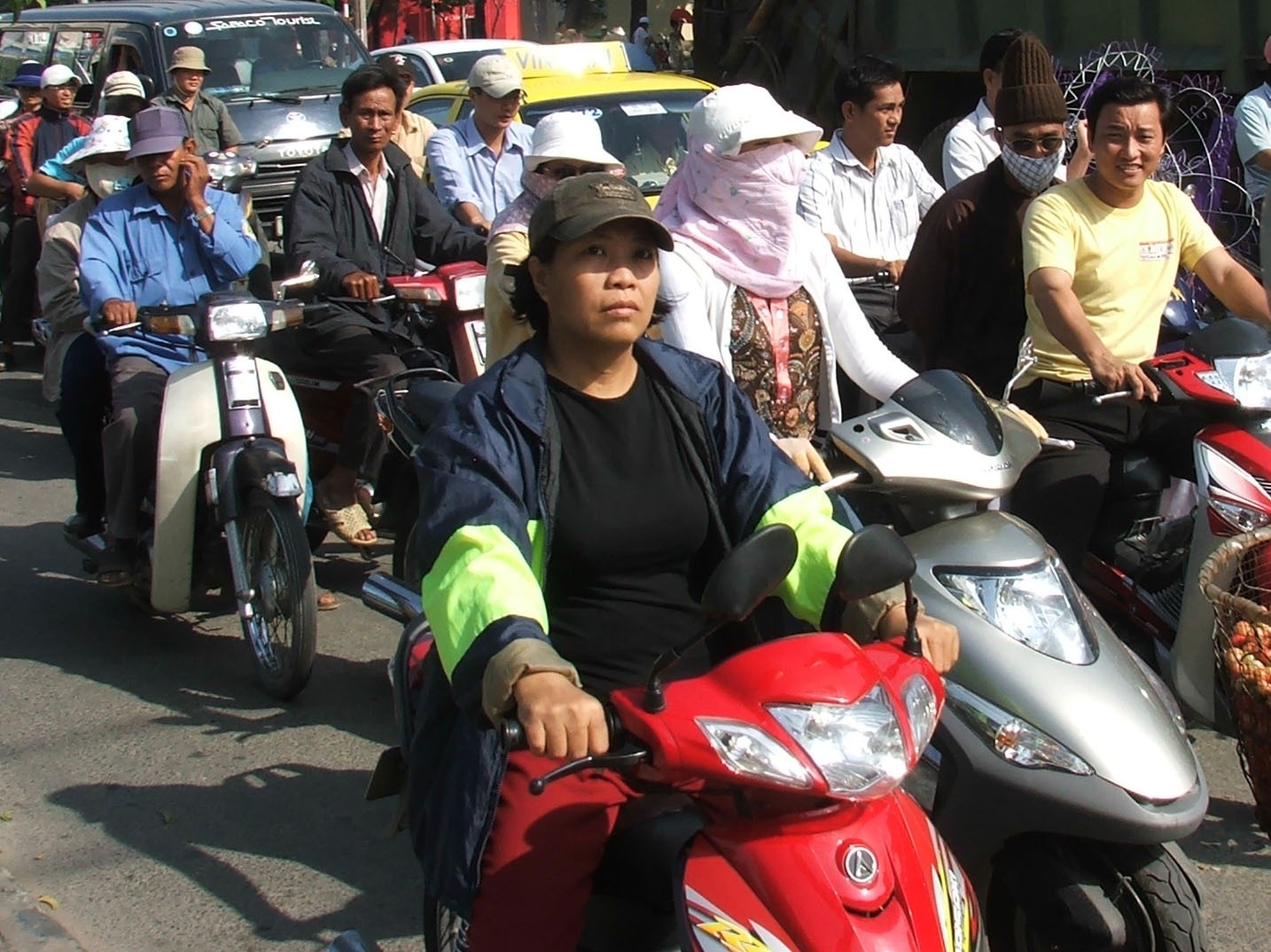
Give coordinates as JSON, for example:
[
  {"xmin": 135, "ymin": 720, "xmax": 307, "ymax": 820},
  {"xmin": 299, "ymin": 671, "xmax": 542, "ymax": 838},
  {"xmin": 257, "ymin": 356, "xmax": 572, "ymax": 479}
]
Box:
[
  {"xmin": 162, "ymin": 45, "xmax": 214, "ymax": 76},
  {"xmin": 517, "ymin": 109, "xmax": 628, "ymax": 181},
  {"xmin": 61, "ymin": 113, "xmax": 134, "ymax": 175},
  {"xmin": 990, "ymin": 29, "xmax": 1069, "ymax": 127},
  {"xmin": 125, "ymin": 106, "xmax": 187, "ymax": 162},
  {"xmin": 101, "ymin": 69, "xmax": 147, "ymax": 102},
  {"xmin": 40, "ymin": 63, "xmax": 85, "ymax": 91},
  {"xmin": 527, "ymin": 169, "xmax": 679, "ymax": 256},
  {"xmin": 466, "ymin": 55, "xmax": 529, "ymax": 103},
  {"xmin": 375, "ymin": 51, "xmax": 420, "ymax": 85},
  {"xmin": 5, "ymin": 59, "xmax": 52, "ymax": 89},
  {"xmin": 681, "ymin": 83, "xmax": 824, "ymax": 155}
]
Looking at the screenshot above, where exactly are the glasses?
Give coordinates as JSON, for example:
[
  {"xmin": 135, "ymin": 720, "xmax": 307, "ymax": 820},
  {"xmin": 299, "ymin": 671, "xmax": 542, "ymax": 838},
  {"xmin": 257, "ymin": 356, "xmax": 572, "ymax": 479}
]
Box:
[
  {"xmin": 491, "ymin": 91, "xmax": 524, "ymax": 102},
  {"xmin": 539, "ymin": 164, "xmax": 602, "ymax": 182},
  {"xmin": 1004, "ymin": 135, "xmax": 1064, "ymax": 155}
]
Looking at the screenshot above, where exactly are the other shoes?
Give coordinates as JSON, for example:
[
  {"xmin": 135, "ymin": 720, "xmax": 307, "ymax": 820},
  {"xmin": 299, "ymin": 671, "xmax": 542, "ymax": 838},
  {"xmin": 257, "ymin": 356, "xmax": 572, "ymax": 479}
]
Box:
[{"xmin": 62, "ymin": 508, "xmax": 102, "ymax": 533}]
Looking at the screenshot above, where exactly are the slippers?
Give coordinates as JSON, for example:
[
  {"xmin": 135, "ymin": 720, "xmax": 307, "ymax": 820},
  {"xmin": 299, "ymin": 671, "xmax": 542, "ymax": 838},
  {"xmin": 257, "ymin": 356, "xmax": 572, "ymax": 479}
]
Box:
[
  {"xmin": 309, "ymin": 482, "xmax": 379, "ymax": 545},
  {"xmin": 97, "ymin": 545, "xmax": 136, "ymax": 588},
  {"xmin": 315, "ymin": 584, "xmax": 342, "ymax": 611}
]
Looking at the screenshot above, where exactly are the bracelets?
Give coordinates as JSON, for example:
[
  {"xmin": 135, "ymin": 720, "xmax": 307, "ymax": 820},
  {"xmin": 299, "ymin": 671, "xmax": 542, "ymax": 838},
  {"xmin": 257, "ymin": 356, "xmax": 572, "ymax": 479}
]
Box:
[{"xmin": 193, "ymin": 206, "xmax": 214, "ymax": 221}]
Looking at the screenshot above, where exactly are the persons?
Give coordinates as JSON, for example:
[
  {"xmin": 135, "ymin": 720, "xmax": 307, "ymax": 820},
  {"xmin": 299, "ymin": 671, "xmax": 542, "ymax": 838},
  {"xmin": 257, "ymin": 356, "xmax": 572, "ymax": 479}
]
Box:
[
  {"xmin": 626, "ymin": 111, "xmax": 689, "ymax": 175},
  {"xmin": 554, "ymin": 17, "xmax": 687, "ymax": 75},
  {"xmin": 796, "ymin": 30, "xmax": 1271, "ymax": 584},
  {"xmin": 0, "ymin": 25, "xmax": 622, "ymax": 609},
  {"xmin": 399, "ymin": 173, "xmax": 960, "ymax": 952},
  {"xmin": 645, "ymin": 85, "xmax": 1051, "ymax": 530}
]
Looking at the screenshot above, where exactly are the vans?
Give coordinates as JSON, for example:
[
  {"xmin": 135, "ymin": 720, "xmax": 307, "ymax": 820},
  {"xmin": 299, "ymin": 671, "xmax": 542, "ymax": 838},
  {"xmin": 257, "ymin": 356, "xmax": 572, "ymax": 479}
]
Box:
[{"xmin": 1, "ymin": 0, "xmax": 379, "ymax": 278}]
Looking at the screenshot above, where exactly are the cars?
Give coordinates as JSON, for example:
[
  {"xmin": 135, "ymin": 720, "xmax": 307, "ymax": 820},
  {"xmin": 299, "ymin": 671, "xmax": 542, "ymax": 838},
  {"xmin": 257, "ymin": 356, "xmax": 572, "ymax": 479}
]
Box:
[
  {"xmin": 342, "ymin": 38, "xmax": 549, "ymax": 98},
  {"xmin": 404, "ymin": 37, "xmax": 831, "ymax": 215}
]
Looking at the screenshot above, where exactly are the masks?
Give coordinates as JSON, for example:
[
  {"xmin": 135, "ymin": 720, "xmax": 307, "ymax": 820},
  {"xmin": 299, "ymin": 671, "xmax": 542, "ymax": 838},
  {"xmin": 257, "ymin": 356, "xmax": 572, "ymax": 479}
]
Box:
[
  {"xmin": 82, "ymin": 158, "xmax": 140, "ymax": 204},
  {"xmin": 1001, "ymin": 140, "xmax": 1067, "ymax": 192}
]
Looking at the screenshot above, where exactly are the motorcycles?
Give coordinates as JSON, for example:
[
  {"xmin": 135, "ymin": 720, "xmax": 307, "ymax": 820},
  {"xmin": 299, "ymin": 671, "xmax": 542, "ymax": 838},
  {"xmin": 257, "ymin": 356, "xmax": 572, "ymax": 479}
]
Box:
[
  {"xmin": 1011, "ymin": 318, "xmax": 1271, "ymax": 849},
  {"xmin": 765, "ymin": 366, "xmax": 1213, "ymax": 952},
  {"xmin": 26, "ymin": 209, "xmax": 1270, "ymax": 705},
  {"xmin": 357, "ymin": 509, "xmax": 993, "ymax": 952}
]
